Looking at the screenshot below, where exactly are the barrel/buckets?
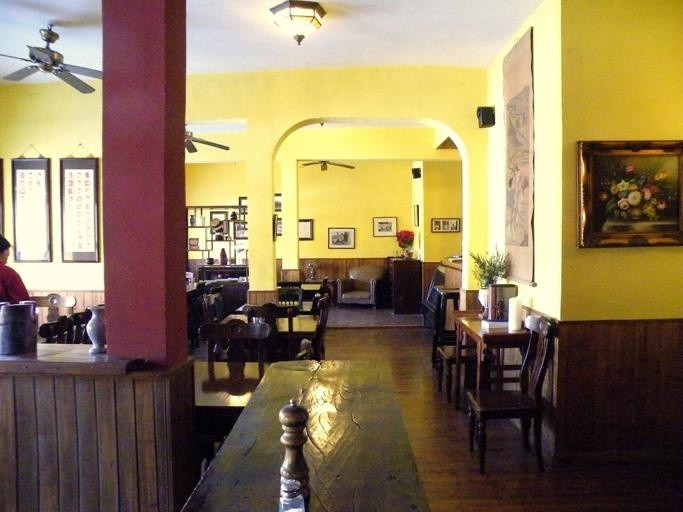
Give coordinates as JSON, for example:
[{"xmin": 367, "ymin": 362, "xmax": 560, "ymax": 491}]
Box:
[{"xmin": 0, "ymin": 300, "xmax": 40, "ymax": 357}]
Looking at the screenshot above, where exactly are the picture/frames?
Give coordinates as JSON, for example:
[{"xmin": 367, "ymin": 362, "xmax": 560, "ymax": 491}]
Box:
[
  {"xmin": 326, "ymin": 227, "xmax": 355, "ymax": 250},
  {"xmin": 576, "ymin": 136, "xmax": 682, "ymax": 249},
  {"xmin": 208, "ymin": 192, "xmax": 316, "ymax": 243},
  {"xmin": 371, "ymin": 216, "xmax": 396, "ymax": 238},
  {"xmin": 430, "ymin": 217, "xmax": 459, "ymax": 234},
  {"xmin": 412, "ymin": 204, "xmax": 419, "ymax": 227},
  {"xmin": 6, "ymin": 155, "xmax": 54, "ymax": 263},
  {"xmin": 56, "ymin": 154, "xmax": 101, "ymax": 264}
]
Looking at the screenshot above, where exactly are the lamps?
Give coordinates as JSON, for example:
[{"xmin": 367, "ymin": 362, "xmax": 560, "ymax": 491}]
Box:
[{"xmin": 269, "ymin": 0, "xmax": 325, "ymax": 49}]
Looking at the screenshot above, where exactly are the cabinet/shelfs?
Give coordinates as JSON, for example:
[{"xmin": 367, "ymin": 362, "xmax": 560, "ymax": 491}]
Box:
[
  {"xmin": 441, "ymin": 259, "xmax": 463, "ymax": 332},
  {"xmin": 420, "ymin": 265, "xmax": 445, "ymax": 327},
  {"xmin": 384, "ymin": 255, "xmax": 422, "ymax": 316}
]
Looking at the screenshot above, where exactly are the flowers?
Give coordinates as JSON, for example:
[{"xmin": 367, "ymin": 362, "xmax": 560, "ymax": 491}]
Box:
[{"xmin": 395, "ymin": 229, "xmax": 414, "ymax": 250}]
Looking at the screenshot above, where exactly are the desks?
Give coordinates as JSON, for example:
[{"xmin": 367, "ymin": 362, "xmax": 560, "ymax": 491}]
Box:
[
  {"xmin": 451, "ymin": 307, "xmax": 539, "ymax": 445},
  {"xmin": 305, "ymin": 277, "xmax": 335, "ymax": 307},
  {"xmin": 193, "ymin": 361, "xmax": 273, "ymax": 407}
]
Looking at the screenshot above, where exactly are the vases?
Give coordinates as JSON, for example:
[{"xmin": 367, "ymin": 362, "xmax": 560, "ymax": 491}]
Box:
[
  {"xmin": 86, "ymin": 306, "xmax": 106, "ymax": 353},
  {"xmin": 305, "ymin": 262, "xmax": 318, "ymax": 279}
]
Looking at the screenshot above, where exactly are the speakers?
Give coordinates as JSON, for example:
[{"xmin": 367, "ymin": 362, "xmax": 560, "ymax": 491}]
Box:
[
  {"xmin": 477, "ymin": 106, "xmax": 494, "ymax": 128},
  {"xmin": 413, "ymin": 168, "xmax": 420, "ymax": 178}
]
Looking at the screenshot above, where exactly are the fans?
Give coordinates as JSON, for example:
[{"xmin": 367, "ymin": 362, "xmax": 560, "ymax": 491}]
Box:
[
  {"xmin": 0, "ymin": 21, "xmax": 103, "ymax": 95},
  {"xmin": 183, "ymin": 123, "xmax": 229, "ymax": 155},
  {"xmin": 301, "ymin": 159, "xmax": 355, "ymax": 172}
]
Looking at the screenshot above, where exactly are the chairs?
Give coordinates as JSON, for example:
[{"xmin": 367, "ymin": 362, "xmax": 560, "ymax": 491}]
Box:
[
  {"xmin": 463, "ymin": 313, "xmax": 555, "ymax": 474},
  {"xmin": 191, "ymin": 278, "xmax": 331, "ymax": 361},
  {"xmin": 333, "ymin": 264, "xmax": 387, "ymax": 311},
  {"xmin": 38, "ymin": 309, "xmax": 93, "ymax": 344},
  {"xmin": 433, "ymin": 289, "xmax": 494, "ymax": 406}
]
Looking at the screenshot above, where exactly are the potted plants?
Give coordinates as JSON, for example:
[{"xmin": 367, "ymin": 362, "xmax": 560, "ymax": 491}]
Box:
[{"xmin": 467, "ymin": 251, "xmax": 514, "ymax": 323}]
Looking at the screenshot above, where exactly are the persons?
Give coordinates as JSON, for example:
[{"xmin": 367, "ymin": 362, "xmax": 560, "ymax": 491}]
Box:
[{"xmin": 0, "ymin": 232, "xmax": 31, "ymax": 305}]
